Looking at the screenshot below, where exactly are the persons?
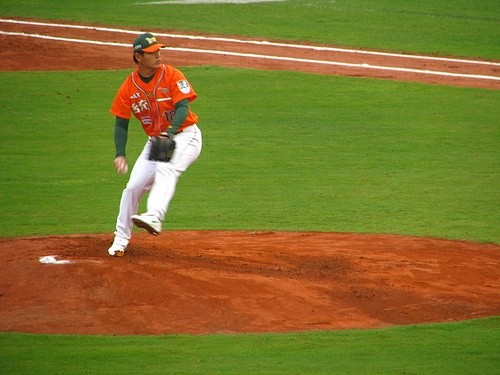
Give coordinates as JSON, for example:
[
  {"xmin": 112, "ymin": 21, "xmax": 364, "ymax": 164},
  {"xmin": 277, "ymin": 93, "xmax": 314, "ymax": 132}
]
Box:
[{"xmin": 108, "ymin": 33, "xmax": 202, "ymax": 257}]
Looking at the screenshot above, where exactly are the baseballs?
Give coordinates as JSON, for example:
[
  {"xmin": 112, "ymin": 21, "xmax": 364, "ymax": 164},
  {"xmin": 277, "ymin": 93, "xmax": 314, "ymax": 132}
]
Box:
[{"xmin": 119, "ymin": 165, "xmax": 128, "ymax": 175}]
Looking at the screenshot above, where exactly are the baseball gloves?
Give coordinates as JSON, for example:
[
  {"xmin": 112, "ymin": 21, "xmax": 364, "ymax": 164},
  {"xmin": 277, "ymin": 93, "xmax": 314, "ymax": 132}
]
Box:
[{"xmin": 150, "ymin": 134, "xmax": 177, "ymax": 166}]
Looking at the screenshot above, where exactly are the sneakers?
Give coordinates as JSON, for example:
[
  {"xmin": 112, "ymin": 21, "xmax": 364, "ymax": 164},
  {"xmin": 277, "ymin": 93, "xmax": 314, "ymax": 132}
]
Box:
[
  {"xmin": 131, "ymin": 214, "xmax": 162, "ymax": 236},
  {"xmin": 108, "ymin": 243, "xmax": 126, "ymax": 257}
]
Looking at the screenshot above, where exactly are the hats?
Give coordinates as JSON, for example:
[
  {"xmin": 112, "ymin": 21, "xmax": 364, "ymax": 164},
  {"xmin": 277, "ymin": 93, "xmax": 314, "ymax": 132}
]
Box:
[{"xmin": 133, "ymin": 33, "xmax": 166, "ymax": 53}]
